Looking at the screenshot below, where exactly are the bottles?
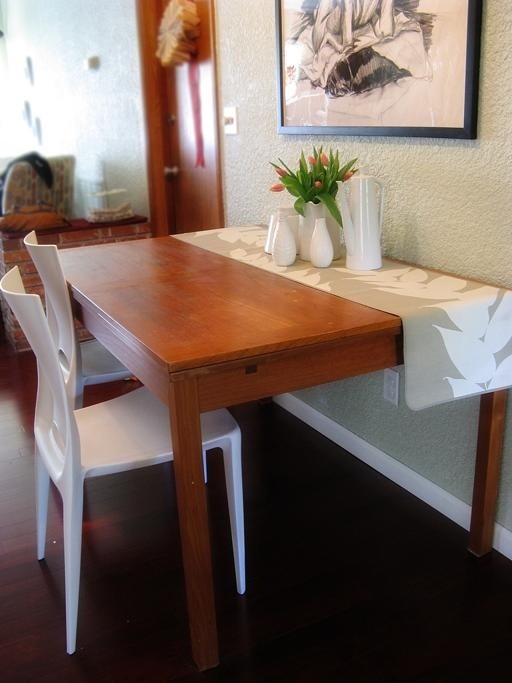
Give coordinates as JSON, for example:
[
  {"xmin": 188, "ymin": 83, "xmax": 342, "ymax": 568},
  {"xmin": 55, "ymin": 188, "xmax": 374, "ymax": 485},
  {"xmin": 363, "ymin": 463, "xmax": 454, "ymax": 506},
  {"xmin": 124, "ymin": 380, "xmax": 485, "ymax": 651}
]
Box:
[
  {"xmin": 310, "ymin": 217, "xmax": 334, "ymax": 269},
  {"xmin": 272, "ymin": 214, "xmax": 295, "ymax": 265}
]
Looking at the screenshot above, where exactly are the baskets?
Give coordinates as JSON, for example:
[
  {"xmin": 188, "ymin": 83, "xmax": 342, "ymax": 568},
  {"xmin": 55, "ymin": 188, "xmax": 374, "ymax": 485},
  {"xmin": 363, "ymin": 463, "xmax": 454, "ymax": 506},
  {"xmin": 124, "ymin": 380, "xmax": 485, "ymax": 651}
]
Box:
[{"xmin": 86, "ymin": 188, "xmax": 135, "ymax": 224}]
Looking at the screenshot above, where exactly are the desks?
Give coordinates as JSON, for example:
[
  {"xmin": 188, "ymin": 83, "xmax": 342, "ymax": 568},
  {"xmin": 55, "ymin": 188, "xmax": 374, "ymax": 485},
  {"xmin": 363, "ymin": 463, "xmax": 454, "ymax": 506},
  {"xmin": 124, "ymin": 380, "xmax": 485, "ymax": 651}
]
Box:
[{"xmin": 57, "ymin": 226, "xmax": 511, "ymax": 672}]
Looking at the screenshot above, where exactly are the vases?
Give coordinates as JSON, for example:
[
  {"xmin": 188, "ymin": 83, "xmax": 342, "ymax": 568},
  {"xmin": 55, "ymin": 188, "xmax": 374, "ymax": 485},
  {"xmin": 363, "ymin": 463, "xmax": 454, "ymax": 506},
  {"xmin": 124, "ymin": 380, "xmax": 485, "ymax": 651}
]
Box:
[{"xmin": 295, "ymin": 199, "xmax": 341, "ymax": 262}]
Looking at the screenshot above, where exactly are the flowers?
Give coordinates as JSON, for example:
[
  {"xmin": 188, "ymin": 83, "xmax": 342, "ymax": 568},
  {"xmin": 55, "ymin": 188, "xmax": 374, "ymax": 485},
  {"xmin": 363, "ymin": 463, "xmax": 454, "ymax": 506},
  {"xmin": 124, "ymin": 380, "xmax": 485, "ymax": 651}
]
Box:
[{"xmin": 268, "ymin": 141, "xmax": 359, "ymax": 226}]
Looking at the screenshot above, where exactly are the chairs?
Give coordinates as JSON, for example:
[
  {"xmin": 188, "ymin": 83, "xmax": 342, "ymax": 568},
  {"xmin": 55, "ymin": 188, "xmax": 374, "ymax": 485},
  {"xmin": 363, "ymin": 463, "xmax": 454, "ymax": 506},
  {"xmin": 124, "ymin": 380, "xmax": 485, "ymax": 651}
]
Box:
[
  {"xmin": 0, "ymin": 266, "xmax": 246, "ymax": 654},
  {"xmin": 23, "ymin": 230, "xmax": 137, "ymax": 410},
  {"xmin": 1, "ymin": 155, "xmax": 76, "ymax": 227}
]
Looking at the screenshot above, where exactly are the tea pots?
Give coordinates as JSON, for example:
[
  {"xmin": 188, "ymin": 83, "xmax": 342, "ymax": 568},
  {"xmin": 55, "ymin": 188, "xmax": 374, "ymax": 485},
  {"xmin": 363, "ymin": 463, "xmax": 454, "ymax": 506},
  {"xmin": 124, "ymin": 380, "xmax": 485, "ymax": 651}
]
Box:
[{"xmin": 336, "ymin": 166, "xmax": 386, "ymax": 273}]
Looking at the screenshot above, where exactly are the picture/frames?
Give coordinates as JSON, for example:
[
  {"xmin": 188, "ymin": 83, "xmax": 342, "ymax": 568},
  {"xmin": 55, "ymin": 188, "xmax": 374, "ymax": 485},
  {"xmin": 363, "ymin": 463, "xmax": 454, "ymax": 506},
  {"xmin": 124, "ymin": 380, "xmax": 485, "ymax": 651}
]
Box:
[{"xmin": 274, "ymin": 0, "xmax": 482, "ymax": 140}]
